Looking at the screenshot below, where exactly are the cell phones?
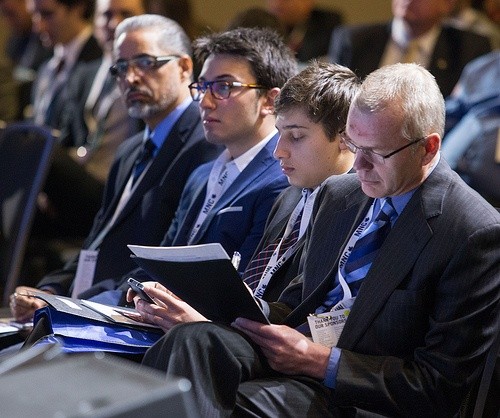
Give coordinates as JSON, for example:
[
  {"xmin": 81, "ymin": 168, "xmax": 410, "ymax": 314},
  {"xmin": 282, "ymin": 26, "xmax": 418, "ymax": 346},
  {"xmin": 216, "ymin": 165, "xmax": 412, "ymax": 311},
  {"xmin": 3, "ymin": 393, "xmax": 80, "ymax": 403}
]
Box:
[{"xmin": 127, "ymin": 278, "xmax": 158, "ymax": 306}]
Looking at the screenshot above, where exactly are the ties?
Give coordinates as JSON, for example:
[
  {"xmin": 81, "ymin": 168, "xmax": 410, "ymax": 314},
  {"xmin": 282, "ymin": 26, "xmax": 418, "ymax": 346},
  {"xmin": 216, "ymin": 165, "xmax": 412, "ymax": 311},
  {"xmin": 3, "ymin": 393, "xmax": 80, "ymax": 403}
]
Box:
[
  {"xmin": 131, "ymin": 138, "xmax": 157, "ymax": 191},
  {"xmin": 325, "ymin": 196, "xmax": 396, "ymax": 314},
  {"xmin": 243, "ymin": 195, "xmax": 309, "ymax": 293},
  {"xmin": 400, "ymin": 41, "xmax": 420, "ymax": 64}
]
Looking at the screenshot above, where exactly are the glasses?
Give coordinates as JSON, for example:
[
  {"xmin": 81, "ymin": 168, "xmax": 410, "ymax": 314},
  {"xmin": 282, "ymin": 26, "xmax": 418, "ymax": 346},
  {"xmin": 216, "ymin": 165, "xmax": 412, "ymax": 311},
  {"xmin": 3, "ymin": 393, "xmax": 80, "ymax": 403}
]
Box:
[
  {"xmin": 338, "ymin": 123, "xmax": 428, "ymax": 166},
  {"xmin": 110, "ymin": 54, "xmax": 181, "ymax": 76},
  {"xmin": 188, "ymin": 80, "xmax": 269, "ymax": 102}
]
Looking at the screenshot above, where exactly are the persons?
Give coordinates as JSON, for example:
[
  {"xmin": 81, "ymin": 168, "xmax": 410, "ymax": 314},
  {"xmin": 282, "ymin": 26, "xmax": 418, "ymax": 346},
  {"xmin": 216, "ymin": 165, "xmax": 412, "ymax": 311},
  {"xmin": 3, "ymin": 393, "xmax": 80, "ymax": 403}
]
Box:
[
  {"xmin": 233, "ymin": 0, "xmax": 500, "ymax": 211},
  {"xmin": 89, "ymin": 29, "xmax": 299, "ymax": 308},
  {"xmin": 22, "ymin": 62, "xmax": 360, "ymax": 365},
  {"xmin": 0, "ymin": 0, "xmax": 163, "ymax": 287},
  {"xmin": 144, "ymin": 61, "xmax": 500, "ymax": 418},
  {"xmin": 1, "ymin": 0, "xmax": 104, "ymax": 159},
  {"xmin": 0, "ymin": 14, "xmax": 226, "ymax": 350},
  {"xmin": 0, "ymin": 0, "xmax": 52, "ymax": 117}
]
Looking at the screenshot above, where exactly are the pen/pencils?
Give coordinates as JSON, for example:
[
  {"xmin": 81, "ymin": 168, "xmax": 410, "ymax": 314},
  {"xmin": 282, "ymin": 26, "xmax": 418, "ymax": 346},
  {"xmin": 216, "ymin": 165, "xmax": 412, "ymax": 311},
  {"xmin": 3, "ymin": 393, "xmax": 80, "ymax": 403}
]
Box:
[{"xmin": 231, "ymin": 251, "xmax": 241, "ymax": 270}]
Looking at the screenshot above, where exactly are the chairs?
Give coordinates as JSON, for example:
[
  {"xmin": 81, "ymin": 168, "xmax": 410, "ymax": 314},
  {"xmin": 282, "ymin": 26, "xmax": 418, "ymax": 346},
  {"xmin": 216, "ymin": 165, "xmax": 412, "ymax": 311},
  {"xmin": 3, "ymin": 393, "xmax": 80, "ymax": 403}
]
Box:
[{"xmin": 0, "ymin": 124, "xmax": 54, "ymax": 306}]
